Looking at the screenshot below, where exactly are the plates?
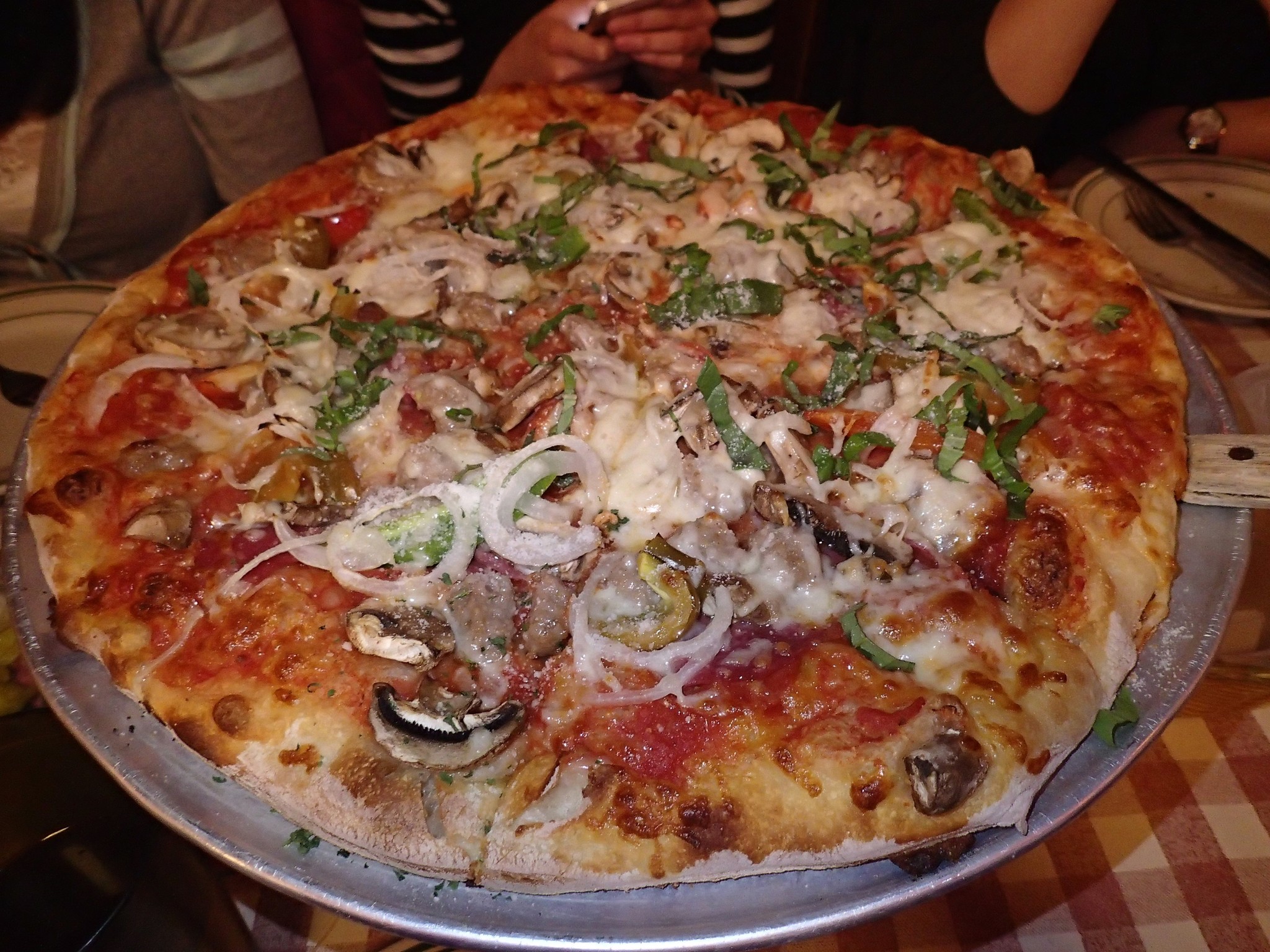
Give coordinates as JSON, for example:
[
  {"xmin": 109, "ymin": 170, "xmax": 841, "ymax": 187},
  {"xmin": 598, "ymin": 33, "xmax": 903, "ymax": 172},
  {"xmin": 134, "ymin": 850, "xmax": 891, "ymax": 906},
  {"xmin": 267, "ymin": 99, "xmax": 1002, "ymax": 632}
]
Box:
[
  {"xmin": 1, "ymin": 283, "xmax": 116, "ymax": 497},
  {"xmin": 1068, "ymin": 155, "xmax": 1270, "ymax": 319}
]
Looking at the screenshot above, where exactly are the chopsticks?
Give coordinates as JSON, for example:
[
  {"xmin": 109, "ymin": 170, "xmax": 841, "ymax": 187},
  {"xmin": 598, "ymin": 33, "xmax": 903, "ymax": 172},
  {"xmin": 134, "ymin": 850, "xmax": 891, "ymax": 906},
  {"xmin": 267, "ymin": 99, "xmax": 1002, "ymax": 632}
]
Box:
[{"xmin": 1084, "ymin": 146, "xmax": 1270, "ymax": 276}]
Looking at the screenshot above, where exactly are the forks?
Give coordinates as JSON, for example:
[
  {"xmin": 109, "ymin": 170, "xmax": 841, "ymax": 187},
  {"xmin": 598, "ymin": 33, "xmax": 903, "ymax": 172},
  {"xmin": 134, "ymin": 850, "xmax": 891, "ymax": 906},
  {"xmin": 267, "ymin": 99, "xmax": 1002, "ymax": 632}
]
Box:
[{"xmin": 1122, "ymin": 185, "xmax": 1270, "ymax": 299}]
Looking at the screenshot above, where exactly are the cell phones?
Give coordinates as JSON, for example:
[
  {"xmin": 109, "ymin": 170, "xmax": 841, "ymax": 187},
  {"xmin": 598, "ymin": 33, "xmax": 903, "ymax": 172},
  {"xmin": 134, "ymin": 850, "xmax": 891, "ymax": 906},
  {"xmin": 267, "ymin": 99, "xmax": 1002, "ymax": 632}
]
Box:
[{"xmin": 583, "ymin": 0, "xmax": 660, "ymax": 38}]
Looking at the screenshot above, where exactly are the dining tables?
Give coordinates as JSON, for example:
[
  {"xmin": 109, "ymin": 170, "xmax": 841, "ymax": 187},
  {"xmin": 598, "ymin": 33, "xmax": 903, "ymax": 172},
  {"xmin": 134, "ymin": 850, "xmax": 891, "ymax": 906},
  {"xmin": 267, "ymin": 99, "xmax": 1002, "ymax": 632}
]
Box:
[{"xmin": 176, "ymin": 108, "xmax": 1270, "ymax": 949}]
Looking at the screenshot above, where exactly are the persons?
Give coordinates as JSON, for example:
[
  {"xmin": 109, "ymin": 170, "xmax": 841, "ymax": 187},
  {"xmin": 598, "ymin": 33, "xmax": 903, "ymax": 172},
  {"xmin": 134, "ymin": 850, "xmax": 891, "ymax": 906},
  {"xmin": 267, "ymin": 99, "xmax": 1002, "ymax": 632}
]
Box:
[
  {"xmin": 0, "ymin": 0, "xmax": 326, "ymax": 292},
  {"xmin": 360, "ymin": 0, "xmax": 1270, "ymax": 187}
]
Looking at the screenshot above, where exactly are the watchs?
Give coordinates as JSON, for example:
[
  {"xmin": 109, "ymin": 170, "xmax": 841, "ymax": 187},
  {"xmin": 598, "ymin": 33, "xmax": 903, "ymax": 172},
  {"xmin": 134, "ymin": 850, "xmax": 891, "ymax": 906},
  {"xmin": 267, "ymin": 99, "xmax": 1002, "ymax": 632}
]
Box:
[{"xmin": 1181, "ymin": 100, "xmax": 1226, "ymax": 156}]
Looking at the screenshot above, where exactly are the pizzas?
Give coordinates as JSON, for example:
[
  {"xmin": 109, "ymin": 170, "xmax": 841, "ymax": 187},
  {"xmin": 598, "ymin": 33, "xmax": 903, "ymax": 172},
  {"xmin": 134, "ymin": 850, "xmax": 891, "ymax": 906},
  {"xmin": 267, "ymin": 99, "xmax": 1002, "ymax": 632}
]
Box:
[{"xmin": 26, "ymin": 77, "xmax": 1192, "ymax": 894}]
